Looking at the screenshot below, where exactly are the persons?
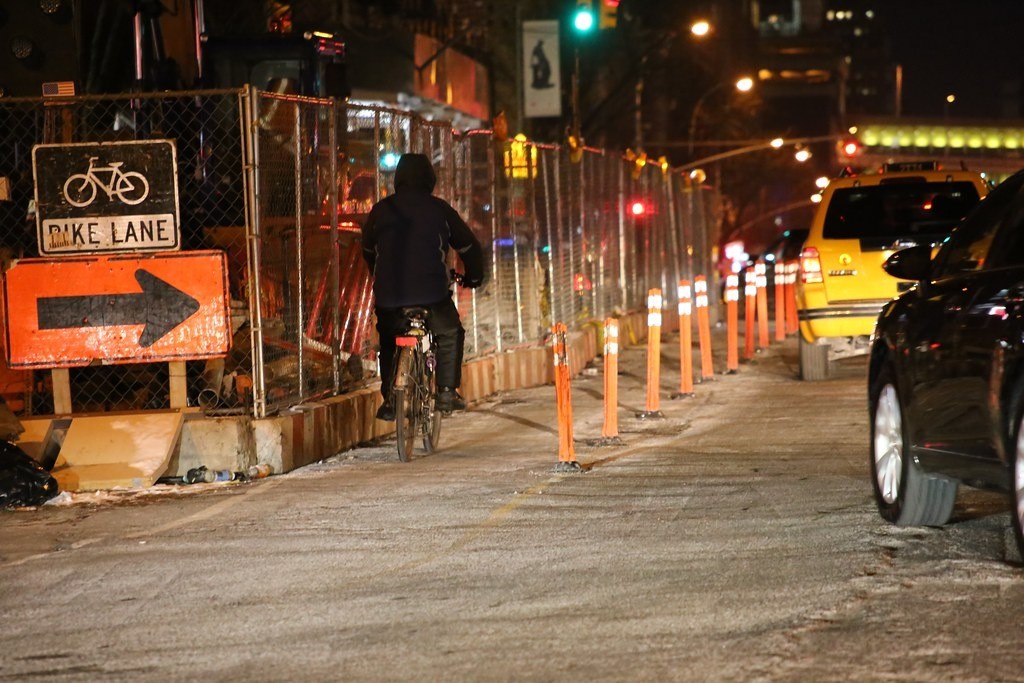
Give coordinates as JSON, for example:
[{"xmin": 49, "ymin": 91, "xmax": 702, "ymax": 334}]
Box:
[
  {"xmin": 126, "ymin": 67, "xmax": 212, "ymax": 409},
  {"xmin": 359, "ymin": 152, "xmax": 484, "ymax": 421}
]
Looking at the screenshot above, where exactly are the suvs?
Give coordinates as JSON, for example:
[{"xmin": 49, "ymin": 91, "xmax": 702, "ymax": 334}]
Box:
[{"xmin": 794, "ymin": 158, "xmax": 1001, "ymax": 382}]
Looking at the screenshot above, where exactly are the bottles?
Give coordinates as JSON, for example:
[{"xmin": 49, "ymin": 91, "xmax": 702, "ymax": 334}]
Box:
[
  {"xmin": 249, "ymin": 465, "xmax": 274, "ymax": 478},
  {"xmin": 204, "ymin": 469, "xmax": 235, "ymax": 483}
]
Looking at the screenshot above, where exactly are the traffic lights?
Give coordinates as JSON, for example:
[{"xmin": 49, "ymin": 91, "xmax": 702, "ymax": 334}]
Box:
[
  {"xmin": 631, "ymin": 203, "xmax": 645, "ymax": 216},
  {"xmin": 575, "ymin": 12, "xmax": 592, "ymax": 30},
  {"xmin": 845, "ymin": 143, "xmax": 856, "ymax": 154},
  {"xmin": 385, "ymin": 155, "xmax": 396, "ymax": 167}
]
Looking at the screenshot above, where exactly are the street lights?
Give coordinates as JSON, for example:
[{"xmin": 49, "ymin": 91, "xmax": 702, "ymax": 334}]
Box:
[
  {"xmin": 634, "ymin": 21, "xmax": 710, "ymax": 156},
  {"xmin": 688, "ymin": 77, "xmax": 754, "ymax": 158}
]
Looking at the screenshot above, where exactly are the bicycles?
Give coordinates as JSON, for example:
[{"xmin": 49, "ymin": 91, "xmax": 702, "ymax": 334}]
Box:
[{"xmin": 387, "ymin": 268, "xmax": 464, "ymax": 463}]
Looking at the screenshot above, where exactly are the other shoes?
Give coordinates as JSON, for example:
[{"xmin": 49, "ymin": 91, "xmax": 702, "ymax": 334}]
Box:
[
  {"xmin": 375, "ymin": 398, "xmax": 395, "ymax": 421},
  {"xmin": 434, "ymin": 386, "xmax": 466, "ymax": 410}
]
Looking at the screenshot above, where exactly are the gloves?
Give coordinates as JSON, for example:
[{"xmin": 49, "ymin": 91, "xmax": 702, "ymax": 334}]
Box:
[{"xmin": 459, "ymin": 269, "xmax": 484, "ymax": 288}]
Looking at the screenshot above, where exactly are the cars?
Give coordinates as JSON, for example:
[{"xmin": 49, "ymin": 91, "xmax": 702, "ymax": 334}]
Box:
[{"xmin": 866, "ymin": 164, "xmax": 1024, "ymax": 553}]
